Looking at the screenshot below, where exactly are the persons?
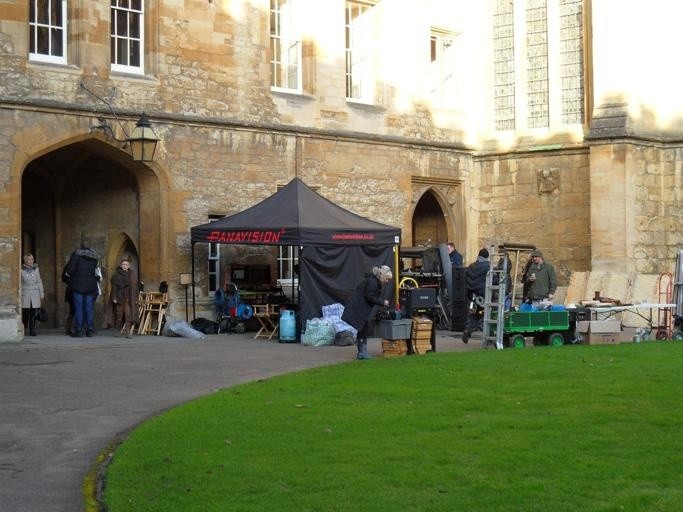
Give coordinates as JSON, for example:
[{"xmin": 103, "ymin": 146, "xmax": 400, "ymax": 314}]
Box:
[
  {"xmin": 62, "ymin": 241, "xmax": 103, "ymax": 338},
  {"xmin": 496, "ymin": 251, "xmax": 511, "ymax": 312},
  {"xmin": 525, "ymin": 251, "xmax": 557, "ymax": 304},
  {"xmin": 21, "ymin": 255, "xmax": 44, "ymax": 336},
  {"xmin": 341, "ymin": 266, "xmax": 392, "ymax": 359},
  {"xmin": 447, "ymin": 243, "xmax": 463, "ymax": 267},
  {"xmin": 110, "ymin": 260, "xmax": 137, "ymax": 332},
  {"xmin": 462, "ymin": 249, "xmax": 498, "ymax": 343}
]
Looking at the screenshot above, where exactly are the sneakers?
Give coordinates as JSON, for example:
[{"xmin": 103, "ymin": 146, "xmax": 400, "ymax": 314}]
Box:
[
  {"xmin": 63, "ymin": 329, "xmax": 93, "ymax": 337},
  {"xmin": 462, "ymin": 330, "xmax": 470, "ymax": 343}
]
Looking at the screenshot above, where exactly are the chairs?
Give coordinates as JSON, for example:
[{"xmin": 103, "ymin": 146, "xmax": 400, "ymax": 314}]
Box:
[
  {"xmin": 121, "ymin": 291, "xmax": 168, "ymax": 335},
  {"xmin": 252, "ymin": 304, "xmax": 280, "ymax": 340}
]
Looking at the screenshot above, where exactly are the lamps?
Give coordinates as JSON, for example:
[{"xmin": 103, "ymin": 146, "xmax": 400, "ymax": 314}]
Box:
[
  {"xmin": 80, "ymin": 83, "xmax": 160, "ymax": 163},
  {"xmin": 179, "ymin": 272, "xmax": 192, "ymax": 322}
]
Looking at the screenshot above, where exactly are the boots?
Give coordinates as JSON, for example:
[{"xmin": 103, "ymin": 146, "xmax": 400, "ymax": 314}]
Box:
[{"xmin": 356, "ymin": 343, "xmax": 373, "ymax": 359}]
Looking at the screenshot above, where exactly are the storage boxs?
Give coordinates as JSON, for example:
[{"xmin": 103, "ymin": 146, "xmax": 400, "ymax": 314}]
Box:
[
  {"xmin": 376, "ymin": 318, "xmax": 412, "ymax": 339},
  {"xmin": 576, "ymin": 320, "xmax": 622, "ymax": 345}
]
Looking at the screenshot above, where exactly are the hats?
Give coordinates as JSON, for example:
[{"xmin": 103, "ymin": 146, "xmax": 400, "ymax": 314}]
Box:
[
  {"xmin": 531, "ymin": 249, "xmax": 543, "ymax": 256},
  {"xmin": 478, "ymin": 248, "xmax": 490, "ymax": 258}
]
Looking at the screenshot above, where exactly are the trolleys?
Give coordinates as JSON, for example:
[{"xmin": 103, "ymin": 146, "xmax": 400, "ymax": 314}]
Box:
[
  {"xmin": 490, "ymin": 305, "xmax": 570, "ymax": 348},
  {"xmin": 220, "ymin": 282, "xmax": 247, "ymax": 334},
  {"xmin": 657, "ymin": 272, "xmax": 672, "ymax": 344}
]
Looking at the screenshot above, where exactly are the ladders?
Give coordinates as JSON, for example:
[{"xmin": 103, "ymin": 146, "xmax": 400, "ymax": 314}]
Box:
[{"xmin": 480, "ymin": 244, "xmax": 509, "ymax": 351}]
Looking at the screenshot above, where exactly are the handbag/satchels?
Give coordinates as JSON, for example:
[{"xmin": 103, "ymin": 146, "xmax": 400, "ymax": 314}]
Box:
[{"xmin": 36, "ymin": 307, "xmax": 49, "ymax": 322}]
